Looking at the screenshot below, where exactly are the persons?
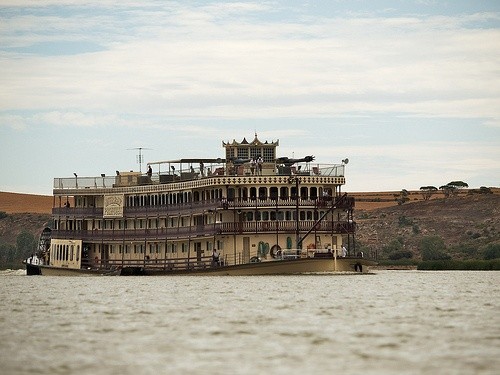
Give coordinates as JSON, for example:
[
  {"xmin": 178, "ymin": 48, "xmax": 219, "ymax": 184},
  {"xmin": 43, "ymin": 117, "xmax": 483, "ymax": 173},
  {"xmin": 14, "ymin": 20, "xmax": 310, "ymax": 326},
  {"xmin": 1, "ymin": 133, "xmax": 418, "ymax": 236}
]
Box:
[
  {"xmin": 161, "ymin": 225, "xmax": 164, "ymax": 234},
  {"xmin": 323, "ymin": 191, "xmax": 330, "ymax": 204},
  {"xmin": 153, "ymin": 253, "xmax": 159, "ymax": 263},
  {"xmin": 345, "ymin": 209, "xmax": 355, "ymax": 228},
  {"xmin": 257, "ymin": 157, "xmax": 264, "ymax": 176},
  {"xmin": 144, "ymin": 227, "xmax": 150, "ymax": 234},
  {"xmin": 190, "ymin": 166, "xmax": 194, "ymax": 173},
  {"xmin": 207, "ymin": 168, "xmax": 213, "ymax": 176},
  {"xmin": 145, "ymin": 253, "xmax": 150, "ymax": 262},
  {"xmin": 339, "ymin": 245, "xmax": 348, "ymax": 258},
  {"xmin": 199, "ymin": 162, "xmax": 204, "ymax": 177},
  {"xmin": 64, "ymin": 201, "xmax": 71, "ymax": 208},
  {"xmin": 94, "ymin": 228, "xmax": 99, "ymax": 235},
  {"xmin": 211, "ymin": 248, "xmax": 220, "ymax": 266},
  {"xmin": 250, "ymin": 157, "xmax": 256, "ymax": 175},
  {"xmin": 146, "ymin": 166, "xmax": 153, "ymax": 180},
  {"xmin": 327, "ymin": 249, "xmax": 334, "ymax": 258},
  {"xmin": 354, "ymin": 249, "xmax": 364, "ymax": 272}
]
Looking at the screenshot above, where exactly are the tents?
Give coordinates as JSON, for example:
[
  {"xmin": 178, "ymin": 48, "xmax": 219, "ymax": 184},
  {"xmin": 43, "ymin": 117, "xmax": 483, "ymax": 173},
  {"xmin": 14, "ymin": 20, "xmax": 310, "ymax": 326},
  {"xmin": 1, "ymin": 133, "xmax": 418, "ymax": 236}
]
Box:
[{"xmin": 147, "ymin": 158, "xmax": 228, "ymax": 163}]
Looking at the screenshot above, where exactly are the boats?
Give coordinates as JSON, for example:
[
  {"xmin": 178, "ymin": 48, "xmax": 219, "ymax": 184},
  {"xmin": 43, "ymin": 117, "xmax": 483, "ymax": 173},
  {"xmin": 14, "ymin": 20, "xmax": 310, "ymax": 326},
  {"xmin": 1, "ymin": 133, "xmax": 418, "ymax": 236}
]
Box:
[{"xmin": 22, "ymin": 131, "xmax": 379, "ymax": 278}]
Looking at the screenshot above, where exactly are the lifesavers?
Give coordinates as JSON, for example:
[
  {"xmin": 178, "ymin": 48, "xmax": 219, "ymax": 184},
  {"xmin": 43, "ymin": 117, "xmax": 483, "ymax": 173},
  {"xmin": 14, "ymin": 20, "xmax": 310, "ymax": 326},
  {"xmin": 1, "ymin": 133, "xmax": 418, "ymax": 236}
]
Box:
[
  {"xmin": 270, "ymin": 245, "xmax": 281, "ymax": 258},
  {"xmin": 95, "ymin": 256, "xmax": 98, "ymax": 263},
  {"xmin": 308, "ymin": 244, "xmax": 315, "ymax": 252}
]
